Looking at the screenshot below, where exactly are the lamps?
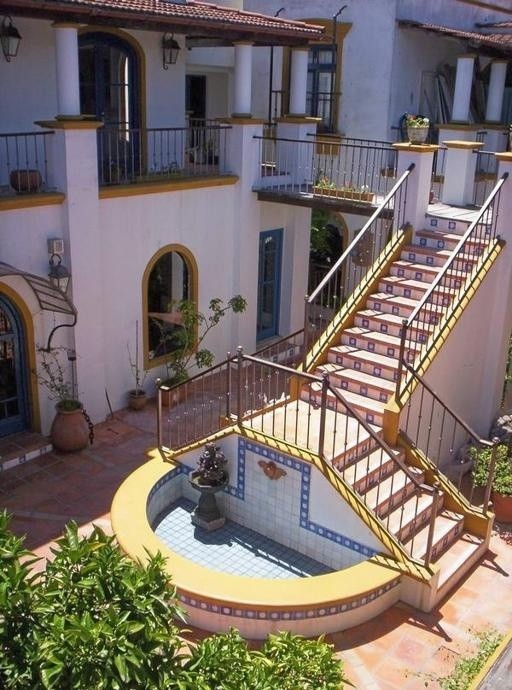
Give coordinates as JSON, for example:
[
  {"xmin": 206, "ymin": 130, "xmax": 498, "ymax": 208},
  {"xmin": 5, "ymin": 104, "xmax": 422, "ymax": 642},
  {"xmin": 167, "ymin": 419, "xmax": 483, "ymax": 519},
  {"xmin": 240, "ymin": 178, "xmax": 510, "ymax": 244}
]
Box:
[
  {"xmin": 49, "ymin": 253, "xmax": 72, "ymax": 294},
  {"xmin": 162, "ymin": 29, "xmax": 181, "ymax": 71},
  {"xmin": 0, "ymin": 14, "xmax": 24, "ymax": 64}
]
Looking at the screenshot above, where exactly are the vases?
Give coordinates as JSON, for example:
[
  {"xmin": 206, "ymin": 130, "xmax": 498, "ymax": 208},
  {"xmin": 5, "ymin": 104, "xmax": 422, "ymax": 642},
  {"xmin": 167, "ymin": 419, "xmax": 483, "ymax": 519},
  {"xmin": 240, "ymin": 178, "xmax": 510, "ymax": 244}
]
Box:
[
  {"xmin": 312, "ymin": 185, "xmax": 374, "ymax": 202},
  {"xmin": 104, "ymin": 165, "xmax": 123, "ymax": 181},
  {"xmin": 407, "ymin": 127, "xmax": 428, "ymax": 144},
  {"xmin": 11, "ymin": 169, "xmax": 42, "ymax": 191}
]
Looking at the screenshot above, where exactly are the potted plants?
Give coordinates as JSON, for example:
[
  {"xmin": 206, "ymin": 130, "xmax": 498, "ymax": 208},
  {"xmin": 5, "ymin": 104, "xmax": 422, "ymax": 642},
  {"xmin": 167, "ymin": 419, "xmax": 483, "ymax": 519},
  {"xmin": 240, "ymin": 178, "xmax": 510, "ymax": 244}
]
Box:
[
  {"xmin": 192, "ymin": 139, "xmax": 213, "ymax": 163},
  {"xmin": 29, "ymin": 340, "xmax": 90, "ymax": 453},
  {"xmin": 467, "ymin": 443, "xmax": 512, "ymax": 523},
  {"xmin": 119, "ymin": 295, "xmax": 248, "ymax": 410},
  {"xmin": 188, "ymin": 440, "xmax": 230, "ymax": 532}
]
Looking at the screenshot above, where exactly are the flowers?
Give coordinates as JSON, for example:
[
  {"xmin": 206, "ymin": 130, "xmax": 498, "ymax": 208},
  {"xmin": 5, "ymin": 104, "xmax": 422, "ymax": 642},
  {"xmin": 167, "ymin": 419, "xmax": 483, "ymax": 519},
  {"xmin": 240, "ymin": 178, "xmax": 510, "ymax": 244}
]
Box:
[
  {"xmin": 317, "ymin": 177, "xmax": 369, "ymax": 193},
  {"xmin": 404, "ymin": 113, "xmax": 430, "ymax": 127}
]
